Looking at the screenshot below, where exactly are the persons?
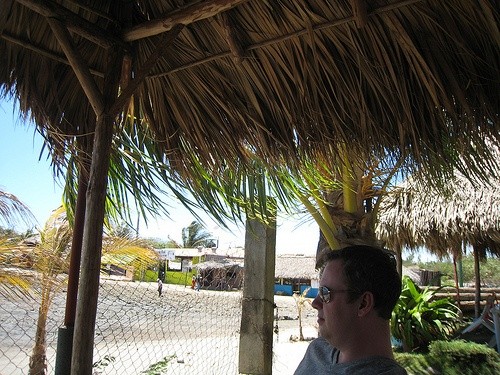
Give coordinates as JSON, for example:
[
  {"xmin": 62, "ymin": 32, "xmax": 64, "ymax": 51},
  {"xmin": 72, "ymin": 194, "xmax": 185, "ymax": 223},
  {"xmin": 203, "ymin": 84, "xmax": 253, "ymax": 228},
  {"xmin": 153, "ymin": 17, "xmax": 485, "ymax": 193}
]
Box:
[
  {"xmin": 191, "ymin": 273, "xmax": 196, "ymax": 289},
  {"xmin": 156, "ymin": 278, "xmax": 163, "ymax": 297},
  {"xmin": 293, "ymin": 245, "xmax": 408, "ymax": 374},
  {"xmin": 195, "ymin": 274, "xmax": 203, "ymax": 291}
]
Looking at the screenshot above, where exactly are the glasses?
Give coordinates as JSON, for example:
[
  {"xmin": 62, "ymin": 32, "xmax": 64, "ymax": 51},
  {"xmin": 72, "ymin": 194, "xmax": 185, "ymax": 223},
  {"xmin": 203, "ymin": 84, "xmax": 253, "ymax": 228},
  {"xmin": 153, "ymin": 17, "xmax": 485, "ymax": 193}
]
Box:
[{"xmin": 314, "ymin": 284, "xmax": 362, "ymax": 305}]
